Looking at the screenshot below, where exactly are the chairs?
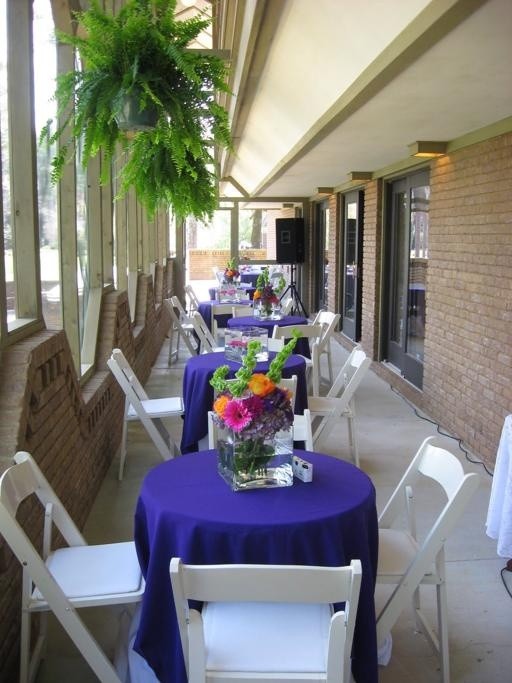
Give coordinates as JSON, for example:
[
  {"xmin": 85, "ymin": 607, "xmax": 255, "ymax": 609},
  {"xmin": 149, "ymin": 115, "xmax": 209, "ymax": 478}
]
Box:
[
  {"xmin": 107, "ymin": 348, "xmax": 186, "ymax": 480},
  {"xmin": 209, "ymin": 303, "xmax": 235, "ymax": 347},
  {"xmin": 164, "ymin": 296, "xmax": 199, "ymax": 365},
  {"xmin": 232, "ymin": 306, "xmax": 254, "ymax": 319},
  {"xmin": 302, "ymin": 344, "xmax": 371, "ymax": 469},
  {"xmin": 209, "ymin": 408, "xmax": 314, "ymax": 454},
  {"xmin": 188, "ymin": 311, "xmax": 226, "ymax": 355},
  {"xmin": 306, "ymin": 308, "xmax": 341, "ymax": 388},
  {"xmin": 171, "ymin": 557, "xmax": 362, "ymax": 683},
  {"xmin": 369, "ymin": 436, "xmax": 478, "ymax": 683},
  {"xmin": 1, "ymin": 451, "xmax": 147, "ymax": 683},
  {"xmin": 272, "ymin": 324, "xmax": 319, "ymax": 398},
  {"xmin": 184, "ymin": 285, "xmax": 200, "ymax": 313}
]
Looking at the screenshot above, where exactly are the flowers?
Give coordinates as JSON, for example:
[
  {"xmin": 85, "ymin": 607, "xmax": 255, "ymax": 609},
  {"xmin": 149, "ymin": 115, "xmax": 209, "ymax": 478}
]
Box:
[
  {"xmin": 211, "ymin": 324, "xmax": 302, "ymax": 475},
  {"xmin": 252, "ymin": 267, "xmax": 285, "ymax": 301},
  {"xmin": 223, "ymin": 257, "xmax": 239, "ymax": 281}
]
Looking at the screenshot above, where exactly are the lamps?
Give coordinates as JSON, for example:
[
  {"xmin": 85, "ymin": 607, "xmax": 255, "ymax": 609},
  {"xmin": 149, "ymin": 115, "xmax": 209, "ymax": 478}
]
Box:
[
  {"xmin": 348, "ymin": 172, "xmax": 375, "ymax": 183},
  {"xmin": 313, "ymin": 187, "xmax": 335, "ymax": 196},
  {"xmin": 407, "ymin": 141, "xmax": 448, "ymax": 158},
  {"xmin": 282, "ymin": 204, "xmax": 294, "ymax": 210}
]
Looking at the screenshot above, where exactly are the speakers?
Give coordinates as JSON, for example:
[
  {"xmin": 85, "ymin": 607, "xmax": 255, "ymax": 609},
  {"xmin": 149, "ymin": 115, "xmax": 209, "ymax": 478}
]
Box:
[{"xmin": 276, "ymin": 218, "xmax": 306, "ymax": 264}]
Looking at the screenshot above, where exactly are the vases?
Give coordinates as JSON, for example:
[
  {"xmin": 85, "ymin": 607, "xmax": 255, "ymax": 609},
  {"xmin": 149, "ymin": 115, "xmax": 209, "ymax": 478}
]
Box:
[
  {"xmin": 217, "ymin": 416, "xmax": 295, "ymax": 492},
  {"xmin": 219, "ymin": 281, "xmax": 241, "ymax": 301},
  {"xmin": 257, "ymin": 298, "xmax": 276, "ymax": 319}
]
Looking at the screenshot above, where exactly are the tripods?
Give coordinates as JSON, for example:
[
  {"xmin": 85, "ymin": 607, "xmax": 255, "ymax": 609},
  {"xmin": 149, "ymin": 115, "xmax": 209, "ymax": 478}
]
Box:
[{"xmin": 279, "ymin": 264, "xmax": 309, "ymax": 319}]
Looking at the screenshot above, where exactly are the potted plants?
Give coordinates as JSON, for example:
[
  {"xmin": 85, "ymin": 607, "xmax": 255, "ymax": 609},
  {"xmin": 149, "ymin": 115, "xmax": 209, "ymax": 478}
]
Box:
[{"xmin": 38, "ymin": 1, "xmax": 237, "ymax": 185}]
